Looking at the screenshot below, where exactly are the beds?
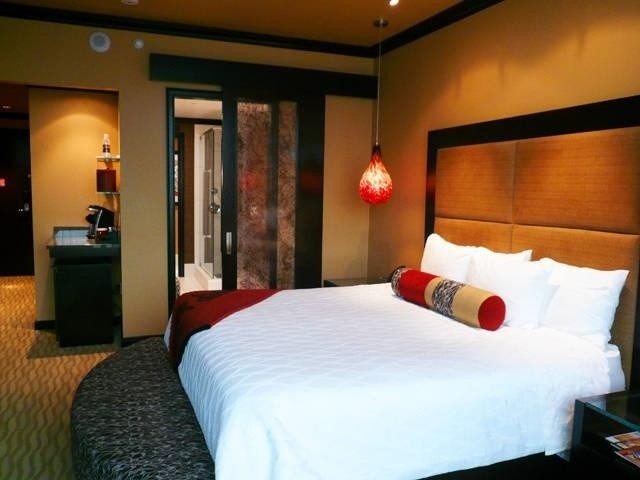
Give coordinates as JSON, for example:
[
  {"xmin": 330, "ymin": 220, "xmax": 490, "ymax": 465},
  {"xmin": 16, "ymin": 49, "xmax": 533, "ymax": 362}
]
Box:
[{"xmin": 162, "ymin": 93, "xmax": 639, "ymax": 480}]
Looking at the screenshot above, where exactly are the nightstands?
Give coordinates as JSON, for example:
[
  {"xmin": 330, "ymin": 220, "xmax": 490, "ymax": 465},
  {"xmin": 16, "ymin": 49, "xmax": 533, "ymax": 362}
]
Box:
[{"xmin": 570, "ymin": 392, "xmax": 640, "ymax": 480}]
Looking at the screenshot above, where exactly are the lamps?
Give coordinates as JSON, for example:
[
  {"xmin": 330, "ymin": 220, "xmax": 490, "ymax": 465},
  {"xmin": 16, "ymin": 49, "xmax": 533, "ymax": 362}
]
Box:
[{"xmin": 356, "ymin": 19, "xmax": 394, "ymax": 207}]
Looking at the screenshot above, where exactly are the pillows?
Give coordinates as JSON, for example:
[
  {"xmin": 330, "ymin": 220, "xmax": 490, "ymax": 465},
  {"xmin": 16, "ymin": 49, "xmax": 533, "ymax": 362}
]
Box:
[
  {"xmin": 420, "ymin": 231, "xmax": 476, "ymax": 283},
  {"xmin": 529, "ymin": 257, "xmax": 630, "ymax": 346},
  {"xmin": 391, "ymin": 267, "xmax": 506, "ymax": 331},
  {"xmin": 504, "ymin": 266, "xmax": 554, "ymax": 325},
  {"xmin": 464, "ymin": 247, "xmax": 537, "ymax": 293}
]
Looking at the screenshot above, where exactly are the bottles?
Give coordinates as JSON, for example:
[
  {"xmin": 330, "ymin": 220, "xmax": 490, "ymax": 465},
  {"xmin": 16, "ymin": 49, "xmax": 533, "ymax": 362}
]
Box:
[{"xmin": 102, "ymin": 134, "xmax": 111, "ymax": 156}]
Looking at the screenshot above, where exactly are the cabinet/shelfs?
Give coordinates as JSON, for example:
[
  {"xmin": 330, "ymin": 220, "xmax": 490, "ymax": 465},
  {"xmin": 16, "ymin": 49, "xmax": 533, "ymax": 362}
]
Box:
[
  {"xmin": 47, "ymin": 226, "xmax": 122, "ymax": 348},
  {"xmin": 95, "ymin": 155, "xmax": 120, "ymax": 196}
]
